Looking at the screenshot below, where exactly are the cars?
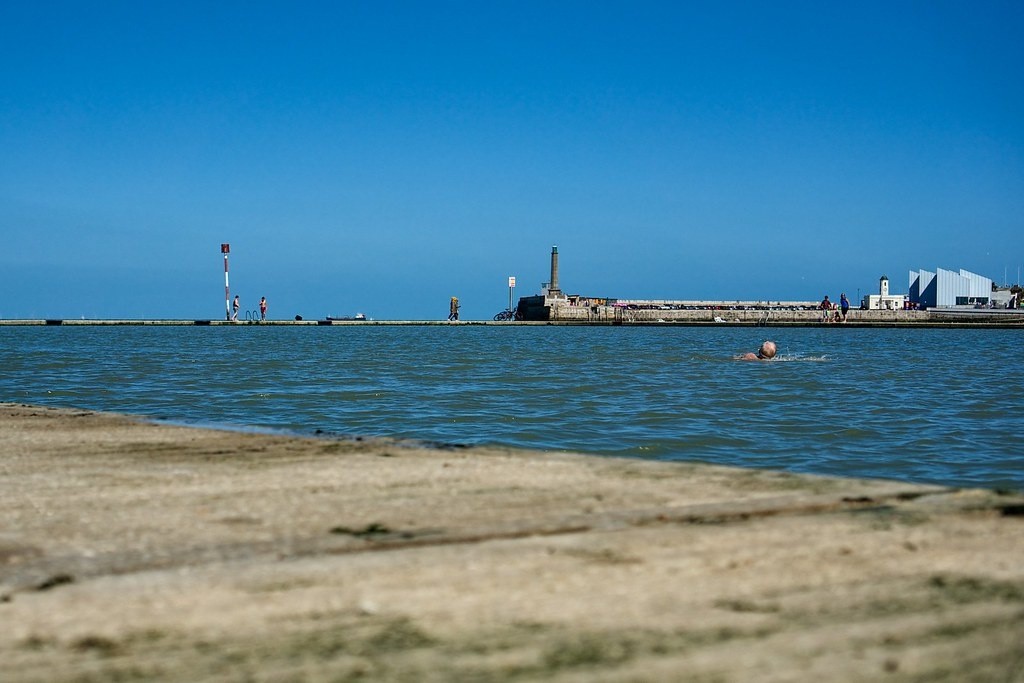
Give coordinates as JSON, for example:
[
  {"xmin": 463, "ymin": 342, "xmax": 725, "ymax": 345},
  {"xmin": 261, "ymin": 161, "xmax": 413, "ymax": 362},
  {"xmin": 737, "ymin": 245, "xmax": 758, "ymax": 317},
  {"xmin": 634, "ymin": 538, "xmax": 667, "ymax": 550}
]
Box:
[{"xmin": 611, "ymin": 302, "xmax": 821, "ymax": 310}]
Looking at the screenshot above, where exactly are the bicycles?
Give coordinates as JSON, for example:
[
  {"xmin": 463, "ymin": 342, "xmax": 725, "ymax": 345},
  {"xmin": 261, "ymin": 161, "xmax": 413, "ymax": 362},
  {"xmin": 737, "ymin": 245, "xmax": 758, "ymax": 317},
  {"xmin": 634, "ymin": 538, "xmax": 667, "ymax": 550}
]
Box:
[{"xmin": 494, "ymin": 308, "xmax": 524, "ymax": 321}]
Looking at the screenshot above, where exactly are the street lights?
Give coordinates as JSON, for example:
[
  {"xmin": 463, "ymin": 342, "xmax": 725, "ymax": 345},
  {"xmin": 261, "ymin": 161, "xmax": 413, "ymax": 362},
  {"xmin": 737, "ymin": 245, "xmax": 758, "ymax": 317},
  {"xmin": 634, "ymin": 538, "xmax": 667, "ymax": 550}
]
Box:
[{"xmin": 220, "ymin": 243, "xmax": 231, "ymax": 321}]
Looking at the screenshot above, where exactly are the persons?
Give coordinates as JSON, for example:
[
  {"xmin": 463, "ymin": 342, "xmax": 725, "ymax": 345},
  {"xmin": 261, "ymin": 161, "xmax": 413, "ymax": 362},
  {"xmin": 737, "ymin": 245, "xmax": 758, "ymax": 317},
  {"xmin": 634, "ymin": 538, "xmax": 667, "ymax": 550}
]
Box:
[
  {"xmin": 1016, "ymin": 295, "xmax": 1021, "ymax": 310},
  {"xmin": 259, "ymin": 297, "xmax": 266, "ymax": 320},
  {"xmin": 744, "ymin": 341, "xmax": 777, "ymax": 359},
  {"xmin": 231, "ymin": 295, "xmax": 239, "ymax": 321},
  {"xmin": 832, "ymin": 312, "xmax": 840, "ymax": 322},
  {"xmin": 840, "ymin": 293, "xmax": 850, "ymax": 322},
  {"xmin": 447, "ymin": 297, "xmax": 461, "ymax": 321},
  {"xmin": 821, "ymin": 296, "xmax": 831, "ymax": 322}
]
